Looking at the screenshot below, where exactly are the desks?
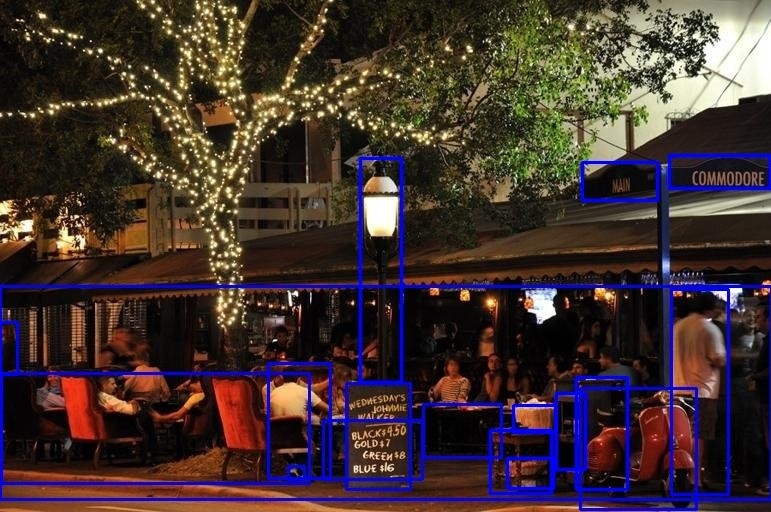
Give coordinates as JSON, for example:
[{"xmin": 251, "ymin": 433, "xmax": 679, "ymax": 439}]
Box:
[
  {"xmin": 423, "ymin": 405, "xmax": 501, "ymax": 458},
  {"xmin": 324, "ymin": 421, "xmax": 423, "ymax": 479}
]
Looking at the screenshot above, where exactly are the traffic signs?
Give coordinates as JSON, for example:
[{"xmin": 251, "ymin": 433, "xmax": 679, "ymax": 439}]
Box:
[
  {"xmin": 669, "ymin": 156, "xmax": 771, "ymax": 189},
  {"xmin": 582, "ymin": 162, "xmax": 657, "ymax": 200}
]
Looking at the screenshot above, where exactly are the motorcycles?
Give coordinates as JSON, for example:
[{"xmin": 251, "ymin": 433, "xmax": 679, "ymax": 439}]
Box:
[{"xmin": 581, "ymin": 388, "xmax": 697, "ymax": 509}]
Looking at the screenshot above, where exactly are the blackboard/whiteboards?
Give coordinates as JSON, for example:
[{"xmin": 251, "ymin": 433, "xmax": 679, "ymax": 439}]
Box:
[{"xmin": 347, "ymin": 384, "xmax": 410, "ymax": 489}]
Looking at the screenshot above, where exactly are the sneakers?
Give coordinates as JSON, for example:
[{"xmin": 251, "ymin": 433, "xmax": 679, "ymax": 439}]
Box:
[{"xmin": 288, "ymin": 466, "xmax": 305, "ymax": 480}]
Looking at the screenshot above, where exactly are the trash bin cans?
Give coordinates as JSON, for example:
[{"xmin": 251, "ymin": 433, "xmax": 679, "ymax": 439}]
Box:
[{"xmin": 576, "ymin": 377, "xmax": 628, "ymax": 490}]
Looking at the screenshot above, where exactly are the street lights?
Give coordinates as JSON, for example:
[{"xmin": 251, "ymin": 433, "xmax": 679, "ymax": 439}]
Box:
[{"xmin": 360, "ymin": 159, "xmax": 402, "ymax": 383}]
